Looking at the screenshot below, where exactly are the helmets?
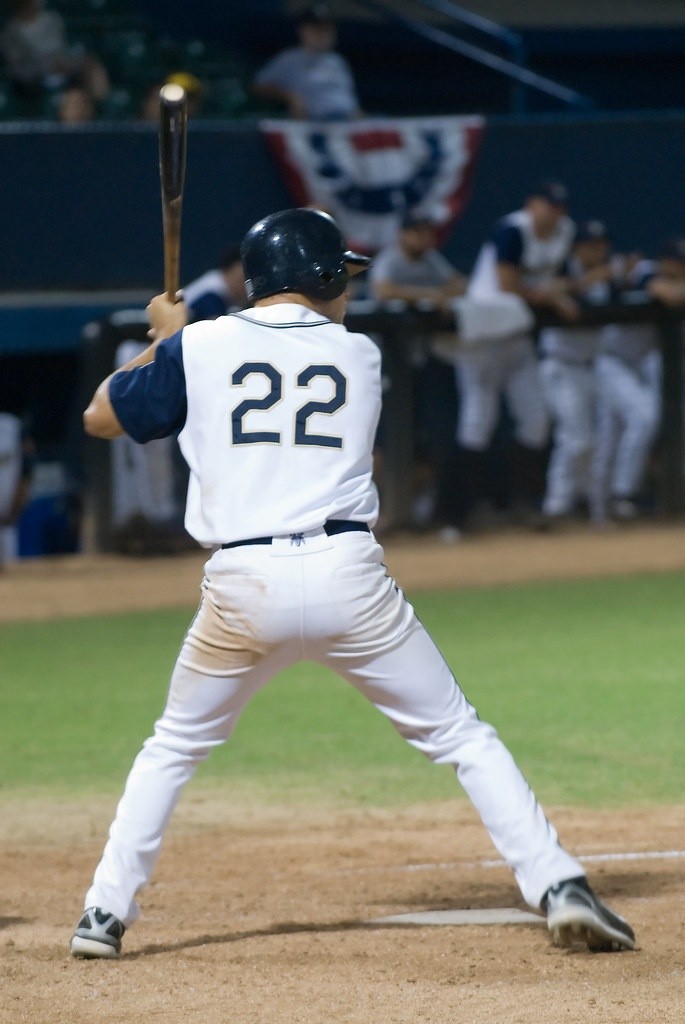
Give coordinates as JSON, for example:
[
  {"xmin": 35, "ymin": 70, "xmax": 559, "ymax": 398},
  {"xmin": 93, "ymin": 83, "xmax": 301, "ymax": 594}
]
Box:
[{"xmin": 242, "ymin": 207, "xmax": 372, "ymax": 298}]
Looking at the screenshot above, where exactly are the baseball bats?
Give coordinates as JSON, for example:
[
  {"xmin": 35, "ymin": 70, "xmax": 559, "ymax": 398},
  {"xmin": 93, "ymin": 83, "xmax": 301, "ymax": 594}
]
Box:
[{"xmin": 158, "ymin": 81, "xmax": 189, "ymax": 301}]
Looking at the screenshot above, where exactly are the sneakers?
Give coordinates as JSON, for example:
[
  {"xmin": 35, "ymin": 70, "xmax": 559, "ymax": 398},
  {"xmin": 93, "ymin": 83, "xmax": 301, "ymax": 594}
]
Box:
[
  {"xmin": 68, "ymin": 906, "xmax": 125, "ymax": 957},
  {"xmin": 542, "ymin": 876, "xmax": 636, "ymax": 952}
]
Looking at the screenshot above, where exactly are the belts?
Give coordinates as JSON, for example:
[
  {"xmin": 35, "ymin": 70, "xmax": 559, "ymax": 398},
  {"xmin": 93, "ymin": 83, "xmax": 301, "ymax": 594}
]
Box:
[{"xmin": 222, "ymin": 520, "xmax": 370, "ymax": 549}]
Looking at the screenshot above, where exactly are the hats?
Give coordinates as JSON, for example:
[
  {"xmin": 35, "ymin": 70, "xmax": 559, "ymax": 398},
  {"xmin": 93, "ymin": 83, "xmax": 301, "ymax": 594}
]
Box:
[
  {"xmin": 531, "ymin": 178, "xmax": 569, "ymax": 206},
  {"xmin": 405, "ymin": 204, "xmax": 454, "ymax": 231}
]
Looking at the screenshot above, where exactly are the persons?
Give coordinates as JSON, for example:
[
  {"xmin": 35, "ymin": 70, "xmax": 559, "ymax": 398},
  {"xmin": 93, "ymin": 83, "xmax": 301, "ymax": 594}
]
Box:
[
  {"xmin": 108, "ymin": 242, "xmax": 245, "ymax": 553},
  {"xmin": 3, "ymin": 0, "xmax": 113, "ymax": 121},
  {"xmin": 0, "ymin": 411, "xmax": 37, "ymax": 559},
  {"xmin": 253, "ymin": 2, "xmax": 367, "ymax": 121},
  {"xmin": 365, "ymin": 190, "xmax": 685, "ymax": 541},
  {"xmin": 71, "ymin": 208, "xmax": 635, "ymax": 959}
]
